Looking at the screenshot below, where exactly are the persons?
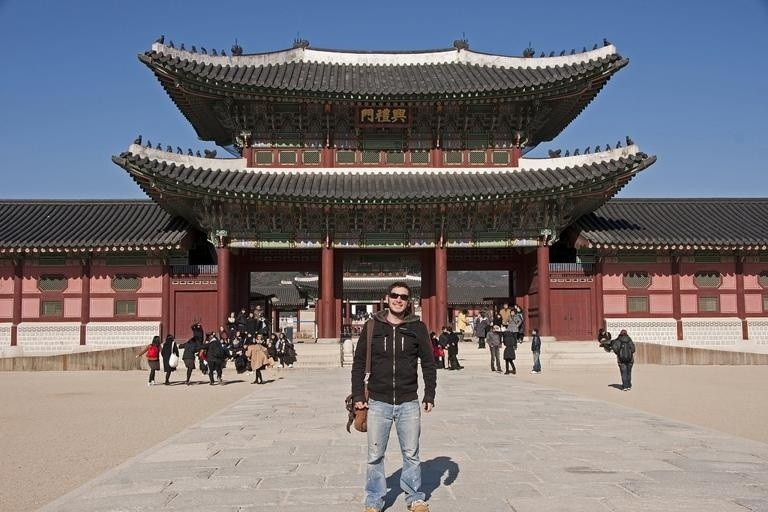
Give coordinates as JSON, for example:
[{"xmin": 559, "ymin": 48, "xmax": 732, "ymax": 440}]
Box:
[
  {"xmin": 351, "ymin": 282, "xmax": 437, "ymax": 512},
  {"xmin": 598, "ymin": 328, "xmax": 635, "ymax": 390},
  {"xmin": 161, "ymin": 334, "xmax": 180, "ymax": 385},
  {"xmin": 431, "ymin": 301, "xmax": 525, "ymax": 375},
  {"xmin": 178, "ymin": 305, "xmax": 295, "ymax": 386},
  {"xmin": 135, "ymin": 336, "xmax": 163, "ymax": 385},
  {"xmin": 531, "ymin": 328, "xmax": 543, "ymax": 375}
]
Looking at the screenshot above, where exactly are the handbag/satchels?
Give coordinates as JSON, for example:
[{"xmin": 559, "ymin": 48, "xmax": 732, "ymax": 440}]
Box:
[
  {"xmin": 433, "ymin": 346, "xmax": 445, "ymax": 357},
  {"xmin": 344, "ymin": 390, "xmax": 369, "ymax": 433},
  {"xmin": 169, "ymin": 341, "xmax": 180, "ymax": 368}
]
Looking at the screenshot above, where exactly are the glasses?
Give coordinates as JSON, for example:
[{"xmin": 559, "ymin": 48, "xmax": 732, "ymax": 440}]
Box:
[{"xmin": 390, "ymin": 293, "xmax": 409, "ymax": 301}]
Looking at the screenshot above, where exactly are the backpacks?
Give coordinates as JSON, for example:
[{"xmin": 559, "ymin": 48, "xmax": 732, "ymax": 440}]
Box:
[
  {"xmin": 147, "ymin": 344, "xmax": 159, "ymax": 358},
  {"xmin": 235, "ymin": 355, "xmax": 246, "ymax": 373},
  {"xmin": 619, "ymin": 342, "xmax": 633, "ymax": 362}
]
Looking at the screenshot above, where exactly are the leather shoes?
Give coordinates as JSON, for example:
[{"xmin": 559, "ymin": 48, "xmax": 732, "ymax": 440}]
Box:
[{"xmin": 251, "ymin": 381, "xmax": 264, "ymax": 385}]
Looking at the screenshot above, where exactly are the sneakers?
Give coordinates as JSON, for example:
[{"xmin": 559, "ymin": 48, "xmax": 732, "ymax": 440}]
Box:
[
  {"xmin": 276, "ymin": 364, "xmax": 284, "ymax": 368},
  {"xmin": 623, "ymin": 387, "xmax": 631, "ymax": 391},
  {"xmin": 492, "ymin": 369, "xmax": 542, "ymax": 375},
  {"xmin": 363, "ymin": 498, "xmax": 387, "ymax": 512},
  {"xmin": 288, "ymin": 363, "xmax": 293, "ymax": 368},
  {"xmin": 407, "ymin": 499, "xmax": 431, "ymax": 512},
  {"xmin": 148, "ymin": 379, "xmax": 225, "ymax": 387}
]
